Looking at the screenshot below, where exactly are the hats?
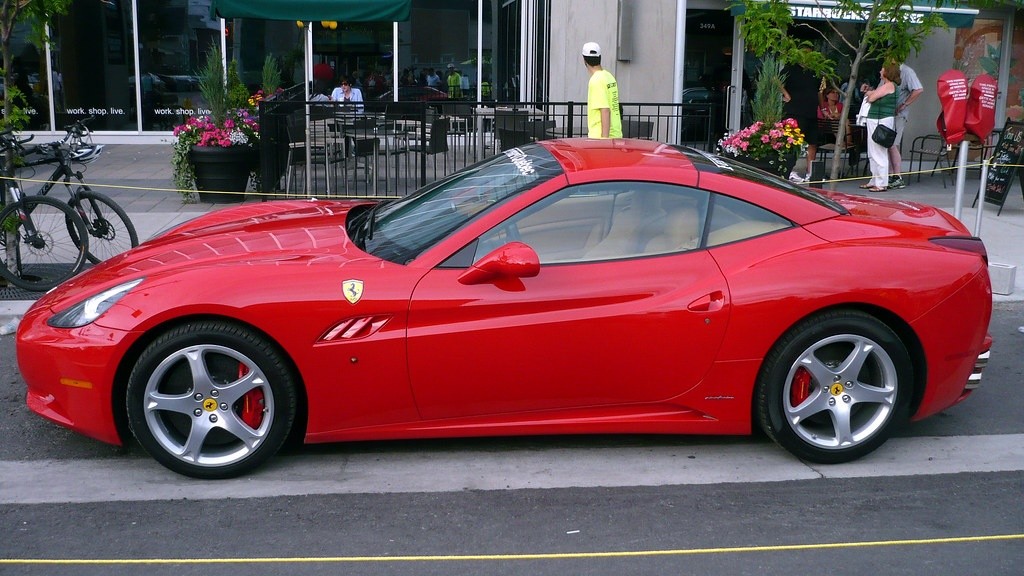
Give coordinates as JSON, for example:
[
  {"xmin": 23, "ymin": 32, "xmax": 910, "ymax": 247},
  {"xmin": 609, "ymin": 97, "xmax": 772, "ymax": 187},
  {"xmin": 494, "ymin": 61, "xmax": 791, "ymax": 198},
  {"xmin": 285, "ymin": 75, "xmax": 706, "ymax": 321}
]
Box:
[
  {"xmin": 447, "ymin": 64, "xmax": 454, "ymax": 68},
  {"xmin": 582, "ymin": 42, "xmax": 601, "ymax": 57}
]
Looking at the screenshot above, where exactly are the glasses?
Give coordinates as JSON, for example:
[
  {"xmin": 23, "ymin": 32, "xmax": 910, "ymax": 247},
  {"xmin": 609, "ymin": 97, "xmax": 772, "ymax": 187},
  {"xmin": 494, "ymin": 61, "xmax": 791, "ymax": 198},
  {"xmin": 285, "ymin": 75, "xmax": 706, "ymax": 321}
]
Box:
[{"xmin": 340, "ymin": 83, "xmax": 348, "ymax": 86}]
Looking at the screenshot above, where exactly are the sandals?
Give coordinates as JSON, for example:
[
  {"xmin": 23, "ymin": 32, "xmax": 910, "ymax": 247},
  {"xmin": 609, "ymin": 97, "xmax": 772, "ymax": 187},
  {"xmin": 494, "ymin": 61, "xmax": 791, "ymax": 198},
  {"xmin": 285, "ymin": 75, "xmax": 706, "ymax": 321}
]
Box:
[{"xmin": 859, "ymin": 183, "xmax": 887, "ymax": 191}]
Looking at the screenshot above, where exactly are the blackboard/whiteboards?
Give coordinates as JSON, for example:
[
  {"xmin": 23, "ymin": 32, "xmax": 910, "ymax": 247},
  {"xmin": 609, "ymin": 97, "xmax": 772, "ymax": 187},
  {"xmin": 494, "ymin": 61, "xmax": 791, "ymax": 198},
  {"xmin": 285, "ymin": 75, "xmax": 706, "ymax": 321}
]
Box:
[{"xmin": 975, "ymin": 117, "xmax": 1024, "ymax": 206}]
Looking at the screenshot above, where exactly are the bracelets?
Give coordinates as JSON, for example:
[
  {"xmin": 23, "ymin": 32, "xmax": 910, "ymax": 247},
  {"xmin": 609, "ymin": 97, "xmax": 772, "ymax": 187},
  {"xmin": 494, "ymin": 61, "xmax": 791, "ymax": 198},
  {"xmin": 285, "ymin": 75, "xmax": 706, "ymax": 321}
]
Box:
[{"xmin": 902, "ymin": 101, "xmax": 908, "ymax": 106}]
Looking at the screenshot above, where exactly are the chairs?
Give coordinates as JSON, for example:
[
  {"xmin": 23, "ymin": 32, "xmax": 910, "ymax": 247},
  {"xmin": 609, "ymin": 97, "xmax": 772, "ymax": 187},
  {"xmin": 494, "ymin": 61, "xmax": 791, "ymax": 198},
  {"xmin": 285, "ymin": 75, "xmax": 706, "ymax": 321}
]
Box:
[
  {"xmin": 622, "ymin": 120, "xmax": 655, "ymax": 140},
  {"xmin": 645, "ymin": 204, "xmax": 698, "ymax": 253},
  {"xmin": 495, "ymin": 110, "xmax": 557, "ymax": 154},
  {"xmin": 908, "ymin": 134, "xmax": 954, "ymax": 189},
  {"xmin": 581, "ymin": 212, "xmax": 646, "ymax": 258},
  {"xmin": 952, "ymin": 140, "xmax": 996, "ymax": 180},
  {"xmin": 281, "ymin": 103, "xmax": 449, "ymax": 198},
  {"xmin": 818, "ymin": 125, "xmax": 859, "ymax": 179}
]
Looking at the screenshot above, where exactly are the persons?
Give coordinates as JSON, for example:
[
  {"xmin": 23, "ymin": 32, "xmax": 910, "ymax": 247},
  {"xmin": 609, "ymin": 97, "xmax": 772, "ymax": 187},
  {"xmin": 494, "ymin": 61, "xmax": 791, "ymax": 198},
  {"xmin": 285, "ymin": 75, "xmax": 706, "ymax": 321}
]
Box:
[
  {"xmin": 52, "ymin": 66, "xmax": 63, "ymax": 112},
  {"xmin": 310, "ymin": 78, "xmax": 334, "ymax": 107},
  {"xmin": 710, "ymin": 48, "xmax": 756, "ymax": 146},
  {"xmin": 582, "ymin": 42, "xmax": 623, "ymax": 138},
  {"xmin": 352, "ymin": 63, "xmax": 491, "ymax": 100},
  {"xmin": 840, "ymin": 74, "xmax": 868, "ymax": 106},
  {"xmin": 331, "ymin": 76, "xmax": 364, "ymax": 111},
  {"xmin": 773, "ymin": 43, "xmax": 923, "ymax": 192}
]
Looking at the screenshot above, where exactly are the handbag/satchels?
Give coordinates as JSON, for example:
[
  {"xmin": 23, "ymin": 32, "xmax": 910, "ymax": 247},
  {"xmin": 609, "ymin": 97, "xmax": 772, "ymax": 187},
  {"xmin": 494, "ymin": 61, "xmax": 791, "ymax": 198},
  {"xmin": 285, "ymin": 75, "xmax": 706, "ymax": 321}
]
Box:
[{"xmin": 872, "ymin": 124, "xmax": 897, "ymax": 148}]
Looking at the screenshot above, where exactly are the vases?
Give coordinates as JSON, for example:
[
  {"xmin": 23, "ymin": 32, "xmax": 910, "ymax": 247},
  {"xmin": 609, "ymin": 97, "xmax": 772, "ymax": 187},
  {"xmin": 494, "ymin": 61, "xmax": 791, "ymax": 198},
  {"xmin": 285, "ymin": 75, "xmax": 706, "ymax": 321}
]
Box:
[
  {"xmin": 721, "ymin": 147, "xmax": 797, "ymax": 181},
  {"xmin": 186, "ymin": 143, "xmax": 252, "ymax": 204}
]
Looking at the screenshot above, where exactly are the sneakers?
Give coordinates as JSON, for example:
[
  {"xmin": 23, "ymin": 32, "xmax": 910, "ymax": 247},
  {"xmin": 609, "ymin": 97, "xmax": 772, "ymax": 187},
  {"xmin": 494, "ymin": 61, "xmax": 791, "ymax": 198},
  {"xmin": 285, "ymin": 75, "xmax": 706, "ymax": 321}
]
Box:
[
  {"xmin": 888, "ymin": 175, "xmax": 905, "ymax": 189},
  {"xmin": 789, "ymin": 171, "xmax": 804, "ymax": 182}
]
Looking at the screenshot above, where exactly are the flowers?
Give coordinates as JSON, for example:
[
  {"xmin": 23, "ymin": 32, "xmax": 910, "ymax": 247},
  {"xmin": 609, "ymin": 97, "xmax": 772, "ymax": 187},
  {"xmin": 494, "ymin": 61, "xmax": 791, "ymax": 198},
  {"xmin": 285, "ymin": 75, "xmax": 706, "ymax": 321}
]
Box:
[
  {"xmin": 715, "ymin": 118, "xmax": 810, "ymax": 179},
  {"xmin": 173, "ymin": 88, "xmax": 285, "ymax": 204}
]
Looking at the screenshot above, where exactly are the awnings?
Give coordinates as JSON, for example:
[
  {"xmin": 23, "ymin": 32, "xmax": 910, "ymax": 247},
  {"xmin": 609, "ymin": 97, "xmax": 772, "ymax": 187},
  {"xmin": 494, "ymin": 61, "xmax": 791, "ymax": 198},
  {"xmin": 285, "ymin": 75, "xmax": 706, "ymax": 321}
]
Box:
[{"xmin": 731, "ymin": 0, "xmax": 979, "ymax": 28}]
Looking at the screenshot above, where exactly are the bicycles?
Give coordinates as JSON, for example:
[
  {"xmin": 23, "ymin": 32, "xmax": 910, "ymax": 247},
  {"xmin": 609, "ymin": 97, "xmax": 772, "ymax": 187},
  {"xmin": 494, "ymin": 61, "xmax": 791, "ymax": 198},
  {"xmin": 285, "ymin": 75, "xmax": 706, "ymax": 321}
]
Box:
[{"xmin": 0, "ymin": 117, "xmax": 139, "ymax": 291}]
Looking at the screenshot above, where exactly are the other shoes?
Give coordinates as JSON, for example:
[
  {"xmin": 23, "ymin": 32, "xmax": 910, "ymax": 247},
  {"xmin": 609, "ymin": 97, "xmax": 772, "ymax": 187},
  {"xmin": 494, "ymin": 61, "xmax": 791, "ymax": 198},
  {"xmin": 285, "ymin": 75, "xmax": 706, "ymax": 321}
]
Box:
[{"xmin": 805, "ymin": 173, "xmax": 810, "ymax": 180}]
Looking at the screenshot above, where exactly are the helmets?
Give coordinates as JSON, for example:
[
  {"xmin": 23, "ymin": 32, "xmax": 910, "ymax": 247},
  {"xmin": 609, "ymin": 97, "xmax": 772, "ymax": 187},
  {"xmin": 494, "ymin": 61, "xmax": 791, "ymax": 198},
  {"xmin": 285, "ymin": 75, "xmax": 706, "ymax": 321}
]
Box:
[{"xmin": 70, "ymin": 144, "xmax": 105, "ymax": 164}]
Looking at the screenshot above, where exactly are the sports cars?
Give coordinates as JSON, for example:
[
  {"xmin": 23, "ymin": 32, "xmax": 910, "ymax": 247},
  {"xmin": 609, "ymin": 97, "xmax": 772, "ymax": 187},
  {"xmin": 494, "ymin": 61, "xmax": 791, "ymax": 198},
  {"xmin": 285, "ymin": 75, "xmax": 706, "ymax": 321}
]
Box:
[
  {"xmin": 17, "ymin": 137, "xmax": 992, "ymax": 482},
  {"xmin": 365, "ymin": 84, "xmax": 447, "ymax": 103}
]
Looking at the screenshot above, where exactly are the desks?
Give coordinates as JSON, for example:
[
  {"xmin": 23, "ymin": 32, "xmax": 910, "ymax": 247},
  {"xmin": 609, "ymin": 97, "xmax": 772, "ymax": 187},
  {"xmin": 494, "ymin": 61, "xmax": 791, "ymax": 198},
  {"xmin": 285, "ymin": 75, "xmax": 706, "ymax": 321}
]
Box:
[
  {"xmin": 475, "ymin": 107, "xmax": 546, "ymax": 161},
  {"xmin": 546, "ymin": 126, "xmax": 587, "ymax": 135}
]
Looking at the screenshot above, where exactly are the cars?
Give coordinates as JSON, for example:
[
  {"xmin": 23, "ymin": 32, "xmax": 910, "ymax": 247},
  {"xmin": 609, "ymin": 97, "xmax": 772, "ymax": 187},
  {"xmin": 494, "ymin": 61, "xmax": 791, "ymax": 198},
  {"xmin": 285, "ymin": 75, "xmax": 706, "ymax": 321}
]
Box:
[{"xmin": 682, "ymin": 88, "xmax": 718, "ymax": 138}]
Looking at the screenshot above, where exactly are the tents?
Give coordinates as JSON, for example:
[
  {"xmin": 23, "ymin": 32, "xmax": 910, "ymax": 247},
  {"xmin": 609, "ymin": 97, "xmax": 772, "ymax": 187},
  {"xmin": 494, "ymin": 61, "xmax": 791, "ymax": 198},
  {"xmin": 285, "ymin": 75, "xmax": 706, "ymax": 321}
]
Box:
[{"xmin": 210, "ymin": 0, "xmax": 411, "ymax": 113}]
